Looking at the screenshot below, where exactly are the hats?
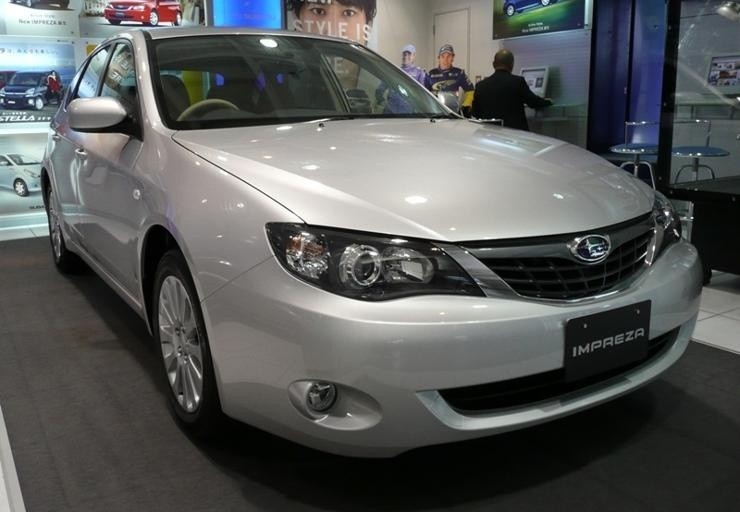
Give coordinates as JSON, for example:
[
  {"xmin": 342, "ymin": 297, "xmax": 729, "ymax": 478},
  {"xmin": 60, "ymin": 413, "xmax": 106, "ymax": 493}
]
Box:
[
  {"xmin": 402, "ymin": 45, "xmax": 416, "ymax": 54},
  {"xmin": 439, "ymin": 44, "xmax": 454, "ymax": 55}
]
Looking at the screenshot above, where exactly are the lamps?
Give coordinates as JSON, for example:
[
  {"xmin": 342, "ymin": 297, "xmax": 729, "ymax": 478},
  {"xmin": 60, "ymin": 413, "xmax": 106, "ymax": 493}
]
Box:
[{"xmin": 716, "ymin": 0, "xmax": 740, "ymax": 22}]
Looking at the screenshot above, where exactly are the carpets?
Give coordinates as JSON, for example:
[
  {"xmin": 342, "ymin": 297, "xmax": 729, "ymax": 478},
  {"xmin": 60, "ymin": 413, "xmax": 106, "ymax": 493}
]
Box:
[{"xmin": 0, "ymin": 235, "xmax": 740, "ymax": 512}]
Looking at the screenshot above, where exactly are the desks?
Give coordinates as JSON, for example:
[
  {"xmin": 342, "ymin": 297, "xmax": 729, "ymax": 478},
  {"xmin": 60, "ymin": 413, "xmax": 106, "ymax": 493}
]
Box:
[
  {"xmin": 674, "ymin": 100, "xmax": 740, "ymax": 120},
  {"xmin": 655, "ymin": 175, "xmax": 739, "ymax": 285},
  {"xmin": 524, "ymin": 104, "xmax": 585, "ymax": 138}
]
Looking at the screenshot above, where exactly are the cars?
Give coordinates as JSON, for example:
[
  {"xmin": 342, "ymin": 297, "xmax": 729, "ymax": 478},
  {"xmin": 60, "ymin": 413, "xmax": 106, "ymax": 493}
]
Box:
[
  {"xmin": 503, "ymin": 0, "xmax": 558, "ymax": 16},
  {"xmin": 104, "ymin": 0, "xmax": 182, "ymax": 25},
  {"xmin": 41, "ymin": 24, "xmax": 705, "ymax": 466},
  {"xmin": 0, "ymin": 152, "xmax": 42, "ymax": 197},
  {"xmin": 0, "ymin": 70, "xmax": 16, "ymax": 88},
  {"xmin": 10, "ymin": 0, "xmax": 69, "ymax": 10},
  {"xmin": 0, "ymin": 69, "xmax": 64, "ymax": 110}
]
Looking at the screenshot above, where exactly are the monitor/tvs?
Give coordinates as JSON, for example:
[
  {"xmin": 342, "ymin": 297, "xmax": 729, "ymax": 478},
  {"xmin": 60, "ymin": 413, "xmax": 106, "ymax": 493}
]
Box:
[{"xmin": 520, "ymin": 66, "xmax": 551, "ymax": 99}]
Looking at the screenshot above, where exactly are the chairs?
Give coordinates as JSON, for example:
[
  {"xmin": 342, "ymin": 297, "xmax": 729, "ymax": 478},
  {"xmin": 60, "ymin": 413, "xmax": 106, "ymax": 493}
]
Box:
[
  {"xmin": 610, "ymin": 119, "xmax": 661, "ymax": 191},
  {"xmin": 159, "ymin": 74, "xmax": 191, "ymax": 121},
  {"xmin": 468, "ymin": 118, "xmax": 505, "ymax": 127},
  {"xmin": 672, "ymin": 119, "xmax": 730, "ymax": 243}
]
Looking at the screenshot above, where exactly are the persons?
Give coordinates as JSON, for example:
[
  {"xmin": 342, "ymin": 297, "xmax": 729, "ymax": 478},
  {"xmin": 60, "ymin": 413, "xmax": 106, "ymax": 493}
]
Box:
[
  {"xmin": 44, "ymin": 70, "xmax": 64, "ymax": 108},
  {"xmin": 471, "ymin": 49, "xmax": 555, "ymax": 132},
  {"xmin": 425, "ymin": 44, "xmax": 474, "ymax": 117},
  {"xmin": 286, "ymin": 0, "xmax": 378, "ymax": 93},
  {"xmin": 374, "ymin": 42, "xmax": 425, "ymax": 114}
]
